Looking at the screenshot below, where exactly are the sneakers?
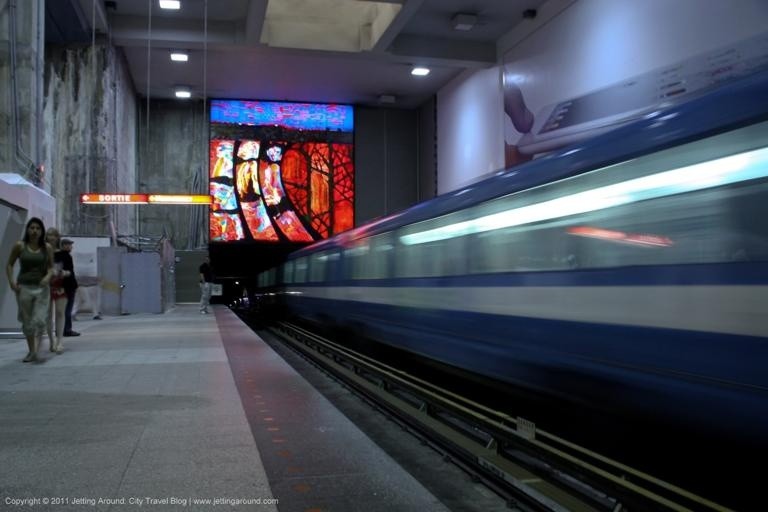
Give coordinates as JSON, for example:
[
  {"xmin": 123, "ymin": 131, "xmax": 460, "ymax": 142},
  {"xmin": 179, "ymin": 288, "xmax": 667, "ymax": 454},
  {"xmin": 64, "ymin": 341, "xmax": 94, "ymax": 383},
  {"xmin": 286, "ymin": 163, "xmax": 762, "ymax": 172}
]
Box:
[
  {"xmin": 22, "ymin": 352, "xmax": 41, "ymax": 363},
  {"xmin": 63, "ymin": 329, "xmax": 81, "ymax": 337},
  {"xmin": 48, "ymin": 345, "xmax": 65, "ymax": 355}
]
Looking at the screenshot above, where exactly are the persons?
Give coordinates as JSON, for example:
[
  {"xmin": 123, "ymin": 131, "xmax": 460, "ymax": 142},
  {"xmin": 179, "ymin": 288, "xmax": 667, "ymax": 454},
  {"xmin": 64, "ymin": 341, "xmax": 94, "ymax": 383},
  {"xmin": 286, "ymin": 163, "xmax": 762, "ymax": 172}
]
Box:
[
  {"xmin": 43, "ymin": 227, "xmax": 74, "ymax": 355},
  {"xmin": 60, "ymin": 237, "xmax": 81, "ymax": 336},
  {"xmin": 501, "ymin": 65, "xmax": 535, "ymax": 170},
  {"xmin": 6, "ymin": 217, "xmax": 54, "ymax": 364},
  {"xmin": 198, "ymin": 256, "xmax": 213, "ymax": 315}
]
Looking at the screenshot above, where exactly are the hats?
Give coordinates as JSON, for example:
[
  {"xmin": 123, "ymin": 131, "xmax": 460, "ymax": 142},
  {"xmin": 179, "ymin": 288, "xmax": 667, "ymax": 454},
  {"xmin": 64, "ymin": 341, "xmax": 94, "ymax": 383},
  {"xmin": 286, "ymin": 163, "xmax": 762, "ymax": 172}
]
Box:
[{"xmin": 61, "ymin": 238, "xmax": 74, "ymax": 245}]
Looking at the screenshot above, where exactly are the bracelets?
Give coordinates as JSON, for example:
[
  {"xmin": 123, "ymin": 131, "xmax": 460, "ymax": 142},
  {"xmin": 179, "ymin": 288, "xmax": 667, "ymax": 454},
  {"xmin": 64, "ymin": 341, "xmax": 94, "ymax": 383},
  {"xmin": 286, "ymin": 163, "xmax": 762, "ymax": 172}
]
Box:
[{"xmin": 65, "ymin": 270, "xmax": 68, "ymax": 278}]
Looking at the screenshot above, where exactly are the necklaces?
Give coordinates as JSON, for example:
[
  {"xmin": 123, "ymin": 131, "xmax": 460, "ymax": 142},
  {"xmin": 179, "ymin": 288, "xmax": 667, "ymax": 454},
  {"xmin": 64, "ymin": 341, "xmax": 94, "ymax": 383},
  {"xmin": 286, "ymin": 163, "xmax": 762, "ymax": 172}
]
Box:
[{"xmin": 28, "ymin": 241, "xmax": 41, "ymax": 252}]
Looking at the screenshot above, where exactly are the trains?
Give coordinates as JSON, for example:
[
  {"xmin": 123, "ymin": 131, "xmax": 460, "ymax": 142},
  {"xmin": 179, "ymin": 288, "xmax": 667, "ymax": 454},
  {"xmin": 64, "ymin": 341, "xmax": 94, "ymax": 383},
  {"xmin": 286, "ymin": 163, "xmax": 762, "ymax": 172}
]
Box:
[{"xmin": 250, "ymin": 73, "xmax": 768, "ymax": 450}]
